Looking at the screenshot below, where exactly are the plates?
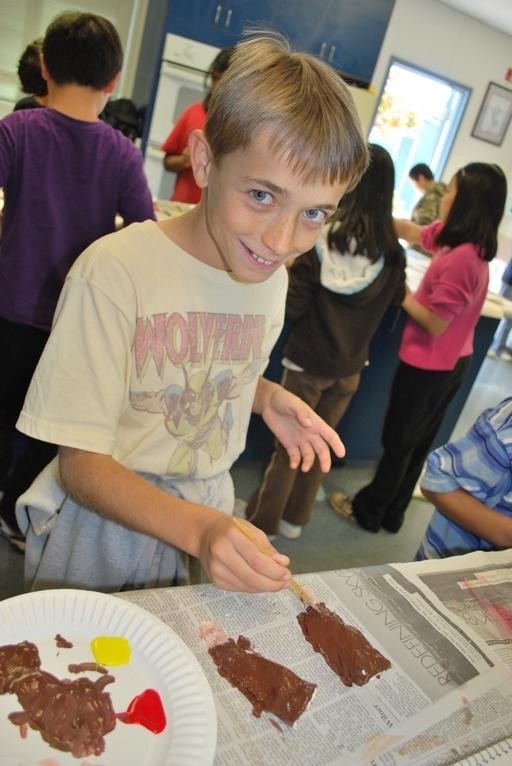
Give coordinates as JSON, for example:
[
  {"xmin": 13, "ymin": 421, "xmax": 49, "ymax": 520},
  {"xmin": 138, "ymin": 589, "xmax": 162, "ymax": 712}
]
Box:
[{"xmin": 0, "ymin": 588, "xmax": 219, "ymax": 766}]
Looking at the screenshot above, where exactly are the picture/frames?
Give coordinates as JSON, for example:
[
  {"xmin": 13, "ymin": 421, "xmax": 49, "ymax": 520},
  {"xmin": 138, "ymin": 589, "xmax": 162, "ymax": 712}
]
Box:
[{"xmin": 469, "ymin": 81, "xmax": 511, "ymax": 146}]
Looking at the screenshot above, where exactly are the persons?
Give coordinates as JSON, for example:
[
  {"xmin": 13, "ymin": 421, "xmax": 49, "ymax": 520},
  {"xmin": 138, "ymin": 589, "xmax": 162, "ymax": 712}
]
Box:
[
  {"xmin": 0, "ymin": 9, "xmax": 157, "ymax": 553},
  {"xmin": 160, "ymin": 44, "xmax": 235, "ymax": 206},
  {"xmin": 14, "ymin": 37, "xmax": 51, "ymax": 109},
  {"xmin": 409, "ymin": 162, "xmax": 451, "ymax": 259},
  {"xmin": 327, "ymin": 160, "xmax": 507, "ymax": 535},
  {"xmin": 14, "ymin": 20, "xmax": 348, "ymax": 602},
  {"xmin": 409, "ymin": 394, "xmax": 512, "ymax": 566},
  {"xmin": 228, "ymin": 141, "xmax": 412, "ymax": 542}
]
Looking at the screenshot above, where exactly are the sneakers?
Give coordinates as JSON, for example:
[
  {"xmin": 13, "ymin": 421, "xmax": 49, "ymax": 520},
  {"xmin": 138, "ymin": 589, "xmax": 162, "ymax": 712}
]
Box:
[
  {"xmin": 233, "ymin": 499, "xmax": 275, "ymax": 541},
  {"xmin": 279, "ymin": 519, "xmax": 301, "ymax": 539},
  {"xmin": 330, "ymin": 494, "xmax": 354, "ymax": 520},
  {"xmin": 0, "ymin": 517, "xmax": 26, "ymax": 551}
]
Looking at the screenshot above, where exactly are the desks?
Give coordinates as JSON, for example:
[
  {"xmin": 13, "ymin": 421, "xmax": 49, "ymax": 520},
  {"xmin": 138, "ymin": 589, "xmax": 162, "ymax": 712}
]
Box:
[
  {"xmin": 152, "ymin": 202, "xmax": 511, "ymax": 467},
  {"xmin": 105, "ymin": 547, "xmax": 511, "ymax": 766}
]
